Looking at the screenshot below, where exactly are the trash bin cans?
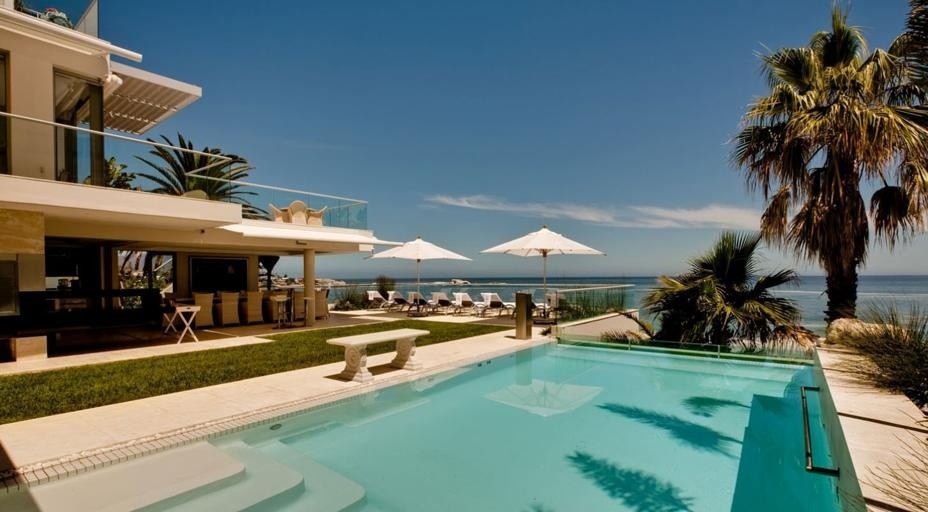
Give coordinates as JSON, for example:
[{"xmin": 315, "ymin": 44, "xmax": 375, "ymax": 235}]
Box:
[{"xmin": 515, "ymin": 293, "xmax": 532, "ymax": 340}]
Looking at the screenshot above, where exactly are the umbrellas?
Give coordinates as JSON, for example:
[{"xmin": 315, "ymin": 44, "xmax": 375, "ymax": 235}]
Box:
[
  {"xmin": 478, "ymin": 224, "xmax": 607, "ymax": 308},
  {"xmin": 363, "ymin": 235, "xmax": 471, "ymax": 310}
]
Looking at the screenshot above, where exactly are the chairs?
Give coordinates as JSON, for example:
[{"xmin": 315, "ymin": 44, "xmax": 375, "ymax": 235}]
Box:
[
  {"xmin": 268, "ymin": 200, "xmax": 330, "ymax": 227},
  {"xmin": 160, "ymin": 285, "xmax": 336, "ymax": 347},
  {"xmin": 363, "ymin": 288, "xmax": 566, "ymax": 320}
]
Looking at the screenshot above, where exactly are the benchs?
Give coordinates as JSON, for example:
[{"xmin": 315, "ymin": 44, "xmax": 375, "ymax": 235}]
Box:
[{"xmin": 327, "ymin": 326, "xmax": 436, "ymax": 383}]
